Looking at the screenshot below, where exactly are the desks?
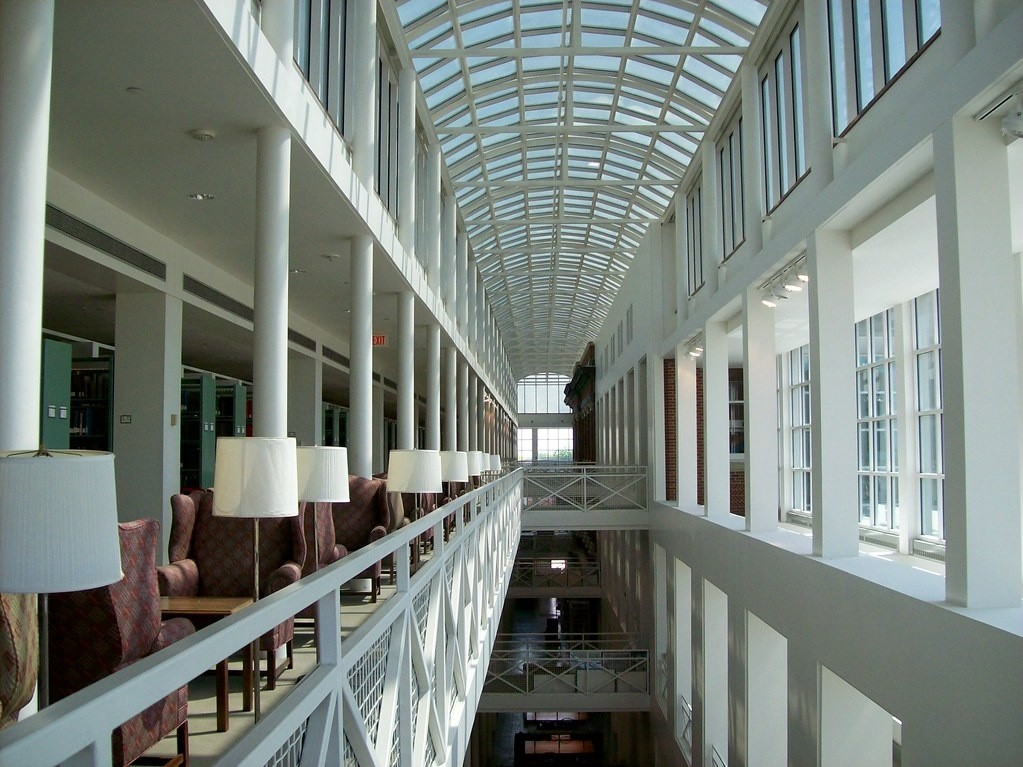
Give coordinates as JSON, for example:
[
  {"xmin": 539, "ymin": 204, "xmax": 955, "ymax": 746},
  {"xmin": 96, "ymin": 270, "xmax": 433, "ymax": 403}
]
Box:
[{"xmin": 159, "ymin": 597, "xmax": 253, "ymax": 732}]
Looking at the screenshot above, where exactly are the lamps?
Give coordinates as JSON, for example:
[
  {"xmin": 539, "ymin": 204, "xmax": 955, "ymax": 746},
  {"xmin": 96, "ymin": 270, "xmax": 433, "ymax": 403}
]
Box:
[
  {"xmin": 482, "ymin": 453, "xmax": 491, "ymax": 471},
  {"xmin": 297, "ymin": 443, "xmax": 350, "ymax": 572},
  {"xmin": 490, "ymin": 454, "xmax": 501, "ymax": 471},
  {"xmin": 439, "ymin": 451, "xmax": 469, "ymax": 534},
  {"xmin": 0, "ymin": 440, "xmax": 124, "ymax": 713},
  {"xmin": 467, "ymin": 450, "xmax": 483, "ymax": 476},
  {"xmin": 211, "ymin": 436, "xmax": 300, "ymax": 723},
  {"xmin": 386, "ymin": 448, "xmax": 443, "ymax": 572}
]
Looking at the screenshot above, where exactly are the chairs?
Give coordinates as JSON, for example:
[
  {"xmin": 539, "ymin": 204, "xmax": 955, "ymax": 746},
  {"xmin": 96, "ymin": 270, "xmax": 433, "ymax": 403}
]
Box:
[
  {"xmin": 155, "ymin": 486, "xmax": 308, "ymax": 689},
  {"xmin": 544, "ymin": 618, "xmax": 558, "ymax": 647},
  {"xmin": 40, "ymin": 518, "xmax": 196, "ymax": 767},
  {"xmin": 300, "ymin": 471, "xmax": 480, "ymax": 603}
]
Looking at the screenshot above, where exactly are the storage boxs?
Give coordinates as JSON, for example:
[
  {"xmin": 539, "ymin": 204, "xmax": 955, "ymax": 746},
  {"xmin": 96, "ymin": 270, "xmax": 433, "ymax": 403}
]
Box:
[
  {"xmin": 533, "ymin": 560, "xmax": 598, "ymax": 587},
  {"xmin": 534, "ymin": 651, "xmax": 648, "ymax": 692}
]
